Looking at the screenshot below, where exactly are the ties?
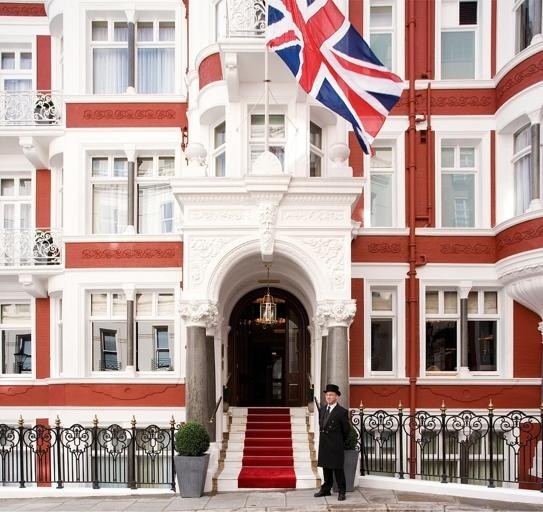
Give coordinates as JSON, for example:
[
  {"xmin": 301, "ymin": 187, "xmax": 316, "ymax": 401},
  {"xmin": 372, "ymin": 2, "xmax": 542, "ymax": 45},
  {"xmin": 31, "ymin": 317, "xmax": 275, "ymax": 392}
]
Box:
[{"xmin": 326, "ymin": 406, "xmax": 331, "ymax": 417}]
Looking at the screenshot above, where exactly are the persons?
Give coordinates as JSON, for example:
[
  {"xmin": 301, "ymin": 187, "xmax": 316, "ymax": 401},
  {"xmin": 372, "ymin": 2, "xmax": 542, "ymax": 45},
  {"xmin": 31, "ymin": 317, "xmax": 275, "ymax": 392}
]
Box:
[{"xmin": 312, "ymin": 384, "xmax": 350, "ymax": 501}]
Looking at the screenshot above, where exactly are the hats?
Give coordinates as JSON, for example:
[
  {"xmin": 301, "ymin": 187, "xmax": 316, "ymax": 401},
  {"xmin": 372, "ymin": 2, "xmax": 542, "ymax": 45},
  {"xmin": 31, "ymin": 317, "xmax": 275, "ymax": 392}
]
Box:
[{"xmin": 322, "ymin": 384, "xmax": 341, "ymax": 397}]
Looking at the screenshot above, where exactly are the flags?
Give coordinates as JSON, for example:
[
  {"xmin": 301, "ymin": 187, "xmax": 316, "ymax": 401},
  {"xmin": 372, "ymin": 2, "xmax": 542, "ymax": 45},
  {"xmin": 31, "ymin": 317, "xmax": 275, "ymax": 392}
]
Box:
[{"xmin": 265, "ymin": 0, "xmax": 405, "ymax": 158}]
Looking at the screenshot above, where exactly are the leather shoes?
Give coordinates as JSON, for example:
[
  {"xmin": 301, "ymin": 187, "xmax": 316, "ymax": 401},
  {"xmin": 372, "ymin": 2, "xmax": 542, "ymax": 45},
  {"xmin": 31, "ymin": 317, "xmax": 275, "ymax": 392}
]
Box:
[
  {"xmin": 314, "ymin": 491, "xmax": 331, "ymax": 497},
  {"xmin": 338, "ymin": 493, "xmax": 346, "ymax": 501}
]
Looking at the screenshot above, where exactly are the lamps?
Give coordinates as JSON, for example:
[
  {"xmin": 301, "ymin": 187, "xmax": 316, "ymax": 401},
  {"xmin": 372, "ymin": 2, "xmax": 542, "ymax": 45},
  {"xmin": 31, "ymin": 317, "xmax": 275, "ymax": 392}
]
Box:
[
  {"xmin": 14, "ymin": 337, "xmax": 30, "ymax": 374},
  {"xmin": 259, "ymin": 262, "xmax": 277, "ymax": 326}
]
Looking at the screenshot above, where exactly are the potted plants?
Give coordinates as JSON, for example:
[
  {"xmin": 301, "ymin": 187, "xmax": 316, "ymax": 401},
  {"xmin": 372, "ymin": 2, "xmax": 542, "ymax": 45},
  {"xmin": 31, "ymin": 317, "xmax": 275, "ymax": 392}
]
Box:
[
  {"xmin": 173, "ymin": 421, "xmax": 210, "ymax": 498},
  {"xmin": 333, "ymin": 425, "xmax": 359, "ymax": 492}
]
